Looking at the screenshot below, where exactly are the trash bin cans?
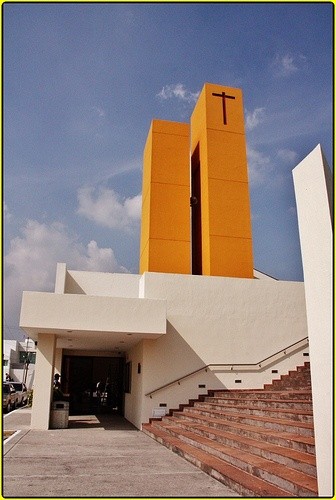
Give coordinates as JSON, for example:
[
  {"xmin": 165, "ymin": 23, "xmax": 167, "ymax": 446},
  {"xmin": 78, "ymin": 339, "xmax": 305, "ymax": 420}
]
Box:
[{"xmin": 51, "ymin": 401, "xmax": 70, "ymax": 430}]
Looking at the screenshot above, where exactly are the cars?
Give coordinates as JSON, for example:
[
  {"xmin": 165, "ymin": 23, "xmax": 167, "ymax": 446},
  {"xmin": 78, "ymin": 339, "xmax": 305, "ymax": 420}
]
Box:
[{"xmin": 3, "ymin": 382, "xmax": 29, "ymax": 413}]
